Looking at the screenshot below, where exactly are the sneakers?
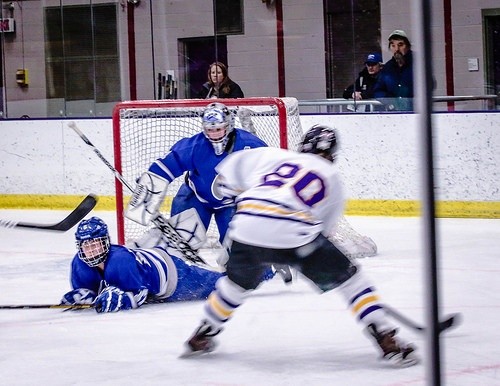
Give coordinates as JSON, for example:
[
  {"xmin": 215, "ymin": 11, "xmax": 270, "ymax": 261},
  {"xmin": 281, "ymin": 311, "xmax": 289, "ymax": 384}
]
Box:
[
  {"xmin": 376, "ymin": 333, "xmax": 421, "ymax": 366},
  {"xmin": 180, "ymin": 319, "xmax": 216, "ymax": 358},
  {"xmin": 273, "ymin": 261, "xmax": 298, "ymax": 283}
]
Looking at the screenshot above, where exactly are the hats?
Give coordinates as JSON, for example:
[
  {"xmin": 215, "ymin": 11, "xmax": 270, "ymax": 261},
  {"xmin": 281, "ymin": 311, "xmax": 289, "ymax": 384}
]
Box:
[
  {"xmin": 364, "ymin": 52, "xmax": 383, "ymax": 63},
  {"xmin": 388, "ymin": 30, "xmax": 407, "ymax": 40}
]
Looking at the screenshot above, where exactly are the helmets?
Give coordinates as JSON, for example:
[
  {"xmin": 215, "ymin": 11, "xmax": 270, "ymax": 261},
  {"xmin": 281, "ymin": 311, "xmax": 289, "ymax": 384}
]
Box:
[
  {"xmin": 202, "ymin": 102, "xmax": 231, "ymax": 133},
  {"xmin": 300, "ymin": 123, "xmax": 337, "ymax": 154},
  {"xmin": 75, "ymin": 216, "xmax": 107, "ymax": 241}
]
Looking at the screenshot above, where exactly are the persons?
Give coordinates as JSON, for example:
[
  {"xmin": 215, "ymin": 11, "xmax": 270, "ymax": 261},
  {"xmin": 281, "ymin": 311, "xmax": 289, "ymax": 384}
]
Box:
[
  {"xmin": 203, "ymin": 62, "xmax": 243, "ymax": 98},
  {"xmin": 180, "ymin": 124, "xmax": 422, "ymax": 367},
  {"xmin": 63, "ymin": 218, "xmax": 291, "ymax": 314},
  {"xmin": 344, "ymin": 51, "xmax": 385, "ymax": 102},
  {"xmin": 130, "ymin": 103, "xmax": 271, "ymax": 263},
  {"xmin": 376, "ymin": 30, "xmax": 438, "ymax": 97}
]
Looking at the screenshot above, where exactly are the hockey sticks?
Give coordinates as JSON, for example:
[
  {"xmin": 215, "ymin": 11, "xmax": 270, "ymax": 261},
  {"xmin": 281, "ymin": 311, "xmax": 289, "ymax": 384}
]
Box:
[
  {"xmin": 0, "ymin": 302, "xmax": 102, "ymax": 310},
  {"xmin": 67, "ymin": 122, "xmax": 212, "ymax": 267},
  {"xmin": 383, "ymin": 304, "xmax": 461, "ymax": 335},
  {"xmin": 157, "ymin": 73, "xmax": 178, "ymax": 100},
  {"xmin": 0, "ymin": 193, "xmax": 99, "ymax": 233}
]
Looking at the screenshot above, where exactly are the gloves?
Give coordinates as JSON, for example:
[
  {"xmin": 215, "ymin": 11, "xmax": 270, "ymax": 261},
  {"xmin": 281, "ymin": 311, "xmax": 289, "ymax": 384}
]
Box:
[
  {"xmin": 61, "ymin": 288, "xmax": 95, "ymax": 306},
  {"xmin": 93, "ymin": 287, "xmax": 138, "ymax": 314}
]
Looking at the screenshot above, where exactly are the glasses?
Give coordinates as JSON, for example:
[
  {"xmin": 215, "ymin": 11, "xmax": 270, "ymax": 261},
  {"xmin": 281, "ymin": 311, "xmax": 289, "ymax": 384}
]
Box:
[{"xmin": 367, "ymin": 62, "xmax": 378, "ymax": 66}]
